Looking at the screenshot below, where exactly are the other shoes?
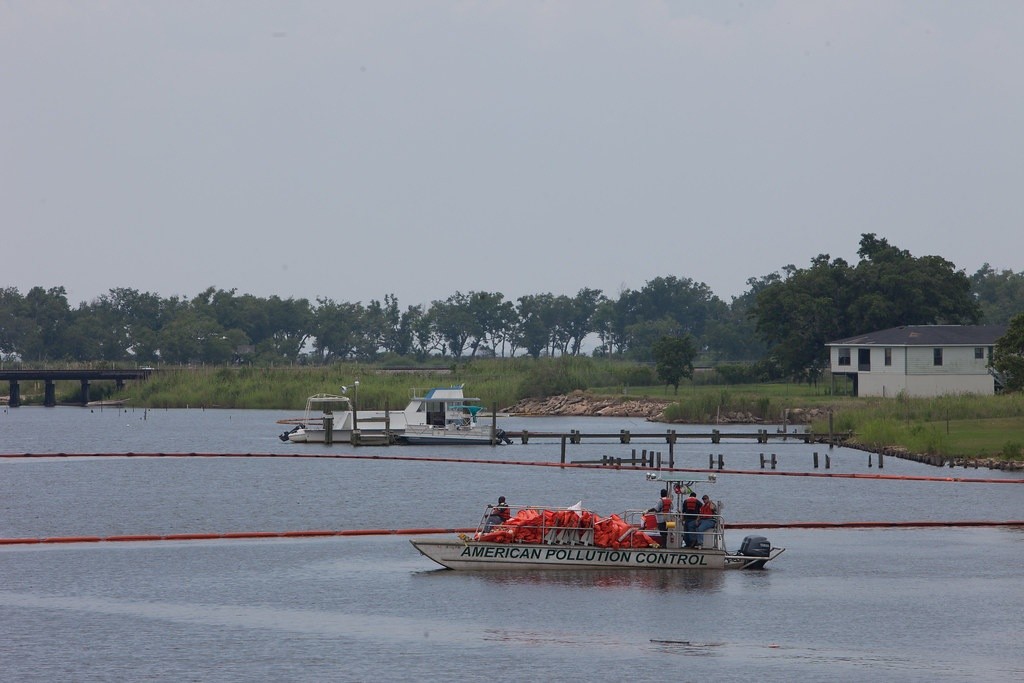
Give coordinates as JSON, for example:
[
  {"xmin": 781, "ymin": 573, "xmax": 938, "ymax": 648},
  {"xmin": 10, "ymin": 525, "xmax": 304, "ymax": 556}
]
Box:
[
  {"xmin": 695, "ymin": 542, "xmax": 703, "ymax": 549},
  {"xmin": 684, "ymin": 542, "xmax": 693, "ymax": 549}
]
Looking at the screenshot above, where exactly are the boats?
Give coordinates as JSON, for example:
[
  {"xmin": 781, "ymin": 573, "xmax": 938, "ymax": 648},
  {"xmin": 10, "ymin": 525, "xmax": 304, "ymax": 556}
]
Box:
[
  {"xmin": 409, "ymin": 471, "xmax": 787, "ymax": 573},
  {"xmin": 278, "ymin": 381, "xmax": 514, "ymax": 447}
]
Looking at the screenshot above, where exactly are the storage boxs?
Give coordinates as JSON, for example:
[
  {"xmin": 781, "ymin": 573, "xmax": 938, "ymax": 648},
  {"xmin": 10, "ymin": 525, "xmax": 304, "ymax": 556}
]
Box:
[{"xmin": 642, "ymin": 515, "xmax": 662, "ymax": 544}]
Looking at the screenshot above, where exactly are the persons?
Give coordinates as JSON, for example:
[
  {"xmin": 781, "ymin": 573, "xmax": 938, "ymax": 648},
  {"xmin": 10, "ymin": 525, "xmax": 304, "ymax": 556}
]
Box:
[
  {"xmin": 483, "ymin": 496, "xmax": 510, "ymax": 533},
  {"xmin": 682, "ymin": 492, "xmax": 717, "ymax": 548},
  {"xmin": 646, "ymin": 489, "xmax": 673, "ymax": 549}
]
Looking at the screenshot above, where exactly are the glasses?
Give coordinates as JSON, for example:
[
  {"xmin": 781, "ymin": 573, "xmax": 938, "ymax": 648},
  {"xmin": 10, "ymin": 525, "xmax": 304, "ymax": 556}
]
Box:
[{"xmin": 702, "ymin": 498, "xmax": 707, "ymax": 500}]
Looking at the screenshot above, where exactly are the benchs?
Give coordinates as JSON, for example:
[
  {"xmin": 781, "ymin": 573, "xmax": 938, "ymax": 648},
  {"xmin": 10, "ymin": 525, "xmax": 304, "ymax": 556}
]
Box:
[{"xmin": 702, "ymin": 528, "xmax": 716, "ymax": 549}]
